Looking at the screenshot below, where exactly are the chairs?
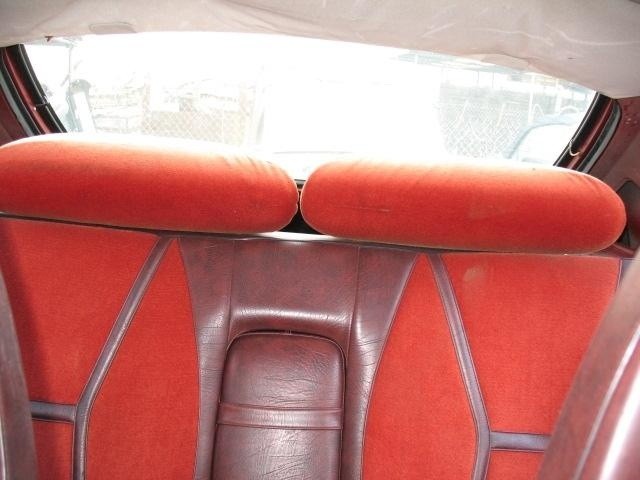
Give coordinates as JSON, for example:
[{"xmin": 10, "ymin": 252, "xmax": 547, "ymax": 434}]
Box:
[
  {"xmin": 299, "ymin": 158, "xmax": 640, "ymax": 479},
  {"xmin": 1, "ymin": 134, "xmax": 298, "ymax": 480}
]
